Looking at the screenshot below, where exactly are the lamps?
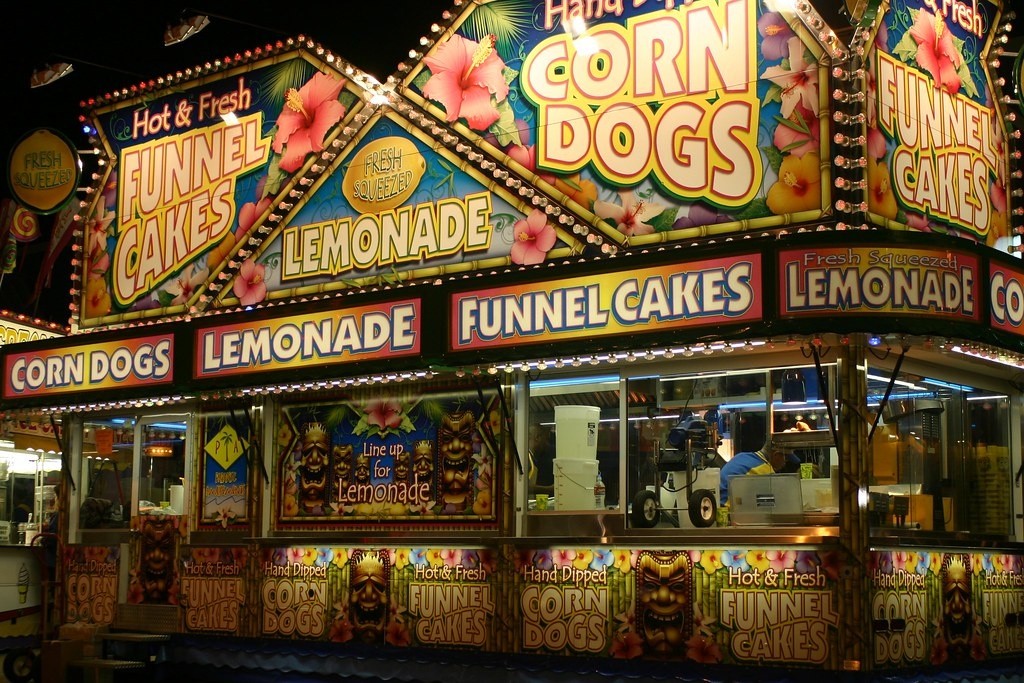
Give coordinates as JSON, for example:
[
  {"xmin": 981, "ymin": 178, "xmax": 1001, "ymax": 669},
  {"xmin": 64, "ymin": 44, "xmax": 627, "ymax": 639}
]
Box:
[
  {"xmin": 31, "ymin": 53, "xmax": 146, "ymax": 89},
  {"xmin": 163, "ymin": 6, "xmax": 294, "ymax": 46}
]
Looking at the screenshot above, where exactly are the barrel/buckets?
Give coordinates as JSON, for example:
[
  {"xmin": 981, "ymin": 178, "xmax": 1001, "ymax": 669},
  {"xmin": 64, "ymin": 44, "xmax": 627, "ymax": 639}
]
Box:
[
  {"xmin": 554, "ymin": 405, "xmax": 600, "ymax": 459},
  {"xmin": 553, "ymin": 459, "xmax": 599, "ymax": 511},
  {"xmin": 168, "ymin": 485, "xmax": 184, "ymax": 514}
]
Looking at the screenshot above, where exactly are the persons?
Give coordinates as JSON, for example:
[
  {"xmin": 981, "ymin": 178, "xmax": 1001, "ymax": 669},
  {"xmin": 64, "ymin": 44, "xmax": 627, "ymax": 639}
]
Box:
[
  {"xmin": 7, "ymin": 484, "xmax": 33, "ymax": 522},
  {"xmin": 528, "ymin": 424, "xmax": 554, "ymax": 500},
  {"xmin": 720, "ymin": 442, "xmax": 786, "ymax": 509},
  {"xmin": 46, "ymin": 483, "xmax": 60, "ymax": 582}
]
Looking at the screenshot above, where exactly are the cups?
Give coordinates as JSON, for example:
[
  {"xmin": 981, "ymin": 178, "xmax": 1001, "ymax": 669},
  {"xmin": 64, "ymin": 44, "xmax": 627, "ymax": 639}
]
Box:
[
  {"xmin": 160, "ymin": 502, "xmax": 169, "ymax": 509},
  {"xmin": 536, "ymin": 494, "xmax": 549, "ymax": 512}
]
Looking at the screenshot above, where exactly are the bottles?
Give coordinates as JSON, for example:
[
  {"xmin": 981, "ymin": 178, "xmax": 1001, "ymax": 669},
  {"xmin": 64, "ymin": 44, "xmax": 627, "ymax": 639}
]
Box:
[
  {"xmin": 26, "ymin": 523, "xmax": 37, "ymax": 545},
  {"xmin": 594, "ymin": 475, "xmax": 605, "ymax": 510}
]
